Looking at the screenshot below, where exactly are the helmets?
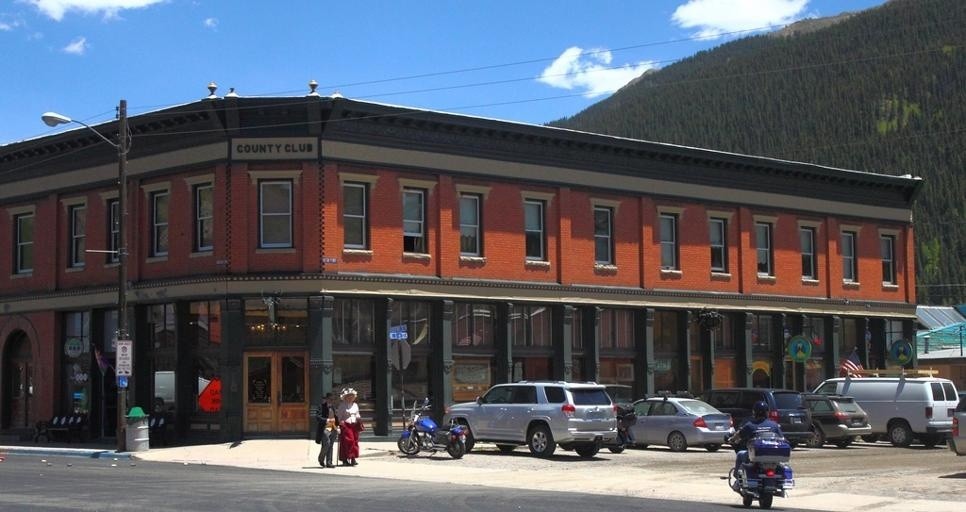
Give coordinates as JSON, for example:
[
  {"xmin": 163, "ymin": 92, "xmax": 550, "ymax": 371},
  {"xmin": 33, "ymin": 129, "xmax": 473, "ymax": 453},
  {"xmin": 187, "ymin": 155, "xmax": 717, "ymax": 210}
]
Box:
[{"xmin": 752, "ymin": 401, "xmax": 769, "ymax": 411}]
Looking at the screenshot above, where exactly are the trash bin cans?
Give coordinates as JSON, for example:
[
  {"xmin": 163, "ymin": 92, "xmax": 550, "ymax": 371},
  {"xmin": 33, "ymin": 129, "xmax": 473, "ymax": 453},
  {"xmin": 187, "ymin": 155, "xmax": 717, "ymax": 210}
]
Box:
[{"xmin": 125, "ymin": 407, "xmax": 150, "ymax": 451}]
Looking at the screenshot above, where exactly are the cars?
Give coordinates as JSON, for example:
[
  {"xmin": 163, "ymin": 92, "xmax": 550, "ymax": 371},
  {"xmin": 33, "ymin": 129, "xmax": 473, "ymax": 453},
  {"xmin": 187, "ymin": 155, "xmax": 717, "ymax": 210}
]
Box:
[
  {"xmin": 809, "ymin": 392, "xmax": 874, "ymax": 446},
  {"xmin": 624, "ymin": 396, "xmax": 742, "ymax": 452}
]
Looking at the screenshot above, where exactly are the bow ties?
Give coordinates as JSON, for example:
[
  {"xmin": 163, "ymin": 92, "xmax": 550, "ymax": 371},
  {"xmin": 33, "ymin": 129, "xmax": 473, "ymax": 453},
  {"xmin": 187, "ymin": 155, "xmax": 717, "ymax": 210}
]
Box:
[{"xmin": 328, "ymin": 405, "xmax": 331, "ymax": 408}]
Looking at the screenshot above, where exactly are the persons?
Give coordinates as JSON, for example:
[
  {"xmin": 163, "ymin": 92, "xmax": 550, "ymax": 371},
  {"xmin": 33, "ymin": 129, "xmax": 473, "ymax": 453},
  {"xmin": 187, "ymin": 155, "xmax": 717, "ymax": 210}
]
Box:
[
  {"xmin": 315, "ymin": 392, "xmax": 338, "ymax": 470},
  {"xmin": 728, "ymin": 401, "xmax": 784, "ymax": 490},
  {"xmin": 338, "ymin": 385, "xmax": 361, "ymax": 467}
]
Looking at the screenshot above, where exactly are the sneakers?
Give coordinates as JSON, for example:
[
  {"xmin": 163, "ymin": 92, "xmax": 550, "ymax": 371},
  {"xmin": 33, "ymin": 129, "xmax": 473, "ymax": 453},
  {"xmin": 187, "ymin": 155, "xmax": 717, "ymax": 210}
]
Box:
[{"xmin": 731, "ymin": 479, "xmax": 743, "ymax": 489}]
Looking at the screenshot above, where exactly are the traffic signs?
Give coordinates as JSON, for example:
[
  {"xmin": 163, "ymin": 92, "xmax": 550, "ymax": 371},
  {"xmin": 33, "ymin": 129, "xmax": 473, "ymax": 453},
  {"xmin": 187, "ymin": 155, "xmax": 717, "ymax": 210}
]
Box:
[{"xmin": 389, "ymin": 322, "xmax": 408, "ymax": 340}]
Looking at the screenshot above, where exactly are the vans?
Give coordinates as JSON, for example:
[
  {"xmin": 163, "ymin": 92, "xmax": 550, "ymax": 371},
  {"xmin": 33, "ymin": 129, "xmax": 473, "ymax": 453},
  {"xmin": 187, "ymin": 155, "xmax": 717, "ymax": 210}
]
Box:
[
  {"xmin": 701, "ymin": 387, "xmax": 812, "ymax": 446},
  {"xmin": 816, "ymin": 376, "xmax": 960, "ymax": 448},
  {"xmin": 951, "ymin": 391, "xmax": 965, "ymax": 454}
]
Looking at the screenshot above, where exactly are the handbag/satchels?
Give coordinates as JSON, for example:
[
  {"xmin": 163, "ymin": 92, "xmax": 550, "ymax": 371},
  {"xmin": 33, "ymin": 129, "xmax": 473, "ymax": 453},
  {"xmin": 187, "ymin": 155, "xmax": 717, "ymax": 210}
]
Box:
[{"xmin": 355, "ymin": 407, "xmax": 366, "ymax": 434}]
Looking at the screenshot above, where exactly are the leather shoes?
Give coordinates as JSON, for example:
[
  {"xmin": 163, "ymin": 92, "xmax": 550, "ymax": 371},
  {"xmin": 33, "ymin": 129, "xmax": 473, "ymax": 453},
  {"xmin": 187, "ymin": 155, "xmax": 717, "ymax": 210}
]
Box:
[
  {"xmin": 343, "ymin": 462, "xmax": 351, "ymax": 466},
  {"xmin": 318, "ymin": 458, "xmax": 325, "ymax": 467},
  {"xmin": 326, "ymin": 464, "xmax": 335, "ymax": 468},
  {"xmin": 351, "ymin": 460, "xmax": 358, "ymax": 466}
]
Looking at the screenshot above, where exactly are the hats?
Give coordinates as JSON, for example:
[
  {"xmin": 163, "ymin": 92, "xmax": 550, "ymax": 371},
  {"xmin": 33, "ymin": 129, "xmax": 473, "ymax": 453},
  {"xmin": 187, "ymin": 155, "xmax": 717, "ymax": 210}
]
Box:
[
  {"xmin": 321, "ymin": 393, "xmax": 338, "ymax": 400},
  {"xmin": 340, "ymin": 387, "xmax": 357, "ymax": 400}
]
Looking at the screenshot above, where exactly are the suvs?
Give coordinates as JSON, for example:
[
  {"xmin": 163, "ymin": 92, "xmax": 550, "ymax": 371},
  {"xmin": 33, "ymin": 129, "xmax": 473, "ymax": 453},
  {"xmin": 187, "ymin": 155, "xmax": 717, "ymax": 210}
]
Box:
[{"xmin": 445, "ymin": 378, "xmax": 623, "ymax": 460}]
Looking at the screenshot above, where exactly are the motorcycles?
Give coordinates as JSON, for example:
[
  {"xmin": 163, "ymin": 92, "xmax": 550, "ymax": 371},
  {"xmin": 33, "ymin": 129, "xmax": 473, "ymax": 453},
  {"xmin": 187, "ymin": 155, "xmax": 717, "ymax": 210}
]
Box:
[
  {"xmin": 397, "ymin": 396, "xmax": 470, "ymax": 459},
  {"xmin": 722, "ymin": 426, "xmax": 797, "ymax": 509}
]
